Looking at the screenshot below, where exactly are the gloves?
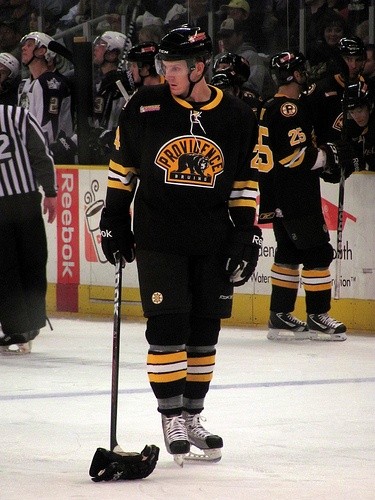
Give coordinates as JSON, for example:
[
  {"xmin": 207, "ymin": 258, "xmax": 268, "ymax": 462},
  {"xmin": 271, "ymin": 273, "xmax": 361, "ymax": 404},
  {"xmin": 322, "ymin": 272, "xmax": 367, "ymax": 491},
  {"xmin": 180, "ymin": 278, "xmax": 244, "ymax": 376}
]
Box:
[
  {"xmin": 309, "ymin": 141, "xmax": 354, "ymax": 176},
  {"xmin": 225, "ymin": 226, "xmax": 263, "ymax": 287},
  {"xmin": 98, "ymin": 129, "xmax": 116, "ymax": 152},
  {"xmin": 48, "ymin": 137, "xmax": 77, "ymax": 156},
  {"xmin": 100, "ymin": 208, "xmax": 136, "ymax": 268}
]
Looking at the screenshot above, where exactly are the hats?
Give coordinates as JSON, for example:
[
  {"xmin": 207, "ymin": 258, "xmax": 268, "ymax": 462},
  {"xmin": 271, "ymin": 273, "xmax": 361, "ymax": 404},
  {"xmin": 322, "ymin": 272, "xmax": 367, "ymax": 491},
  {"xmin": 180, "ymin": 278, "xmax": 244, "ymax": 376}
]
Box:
[
  {"xmin": 326, "ymin": 15, "xmax": 345, "ymax": 27},
  {"xmin": 222, "ymin": 0, "xmax": 250, "ymax": 13},
  {"xmin": 217, "ymin": 18, "xmax": 244, "ymax": 33}
]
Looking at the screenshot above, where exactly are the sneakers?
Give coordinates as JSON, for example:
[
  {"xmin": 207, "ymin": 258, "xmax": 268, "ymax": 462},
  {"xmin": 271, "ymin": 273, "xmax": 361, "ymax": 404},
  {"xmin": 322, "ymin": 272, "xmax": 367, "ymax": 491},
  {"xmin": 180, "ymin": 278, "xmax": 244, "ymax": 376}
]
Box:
[
  {"xmin": 182, "ymin": 407, "xmax": 223, "ymax": 461},
  {"xmin": 307, "ymin": 313, "xmax": 347, "ymax": 341},
  {"xmin": 0, "ymin": 327, "xmax": 40, "ymax": 354},
  {"xmin": 267, "ymin": 311, "xmax": 309, "ymax": 340},
  {"xmin": 157, "ymin": 407, "xmax": 190, "ymax": 468}
]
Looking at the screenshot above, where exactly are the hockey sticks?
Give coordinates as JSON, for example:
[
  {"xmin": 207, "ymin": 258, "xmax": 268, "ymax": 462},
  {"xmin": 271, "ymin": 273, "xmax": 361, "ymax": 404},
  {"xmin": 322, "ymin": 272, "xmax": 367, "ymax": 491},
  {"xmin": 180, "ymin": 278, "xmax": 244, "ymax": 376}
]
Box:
[
  {"xmin": 86, "ymin": 248, "xmax": 162, "ymax": 482},
  {"xmin": 335, "ymin": 167, "xmax": 344, "ymax": 304}
]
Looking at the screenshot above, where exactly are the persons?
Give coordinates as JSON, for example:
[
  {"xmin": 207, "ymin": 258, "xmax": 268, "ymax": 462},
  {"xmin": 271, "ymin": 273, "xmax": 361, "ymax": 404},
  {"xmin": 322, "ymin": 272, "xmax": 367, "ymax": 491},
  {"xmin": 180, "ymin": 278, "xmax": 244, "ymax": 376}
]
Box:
[
  {"xmin": 18, "ymin": 31, "xmax": 76, "ymax": 165},
  {"xmin": 99, "ymin": 24, "xmax": 262, "ymax": 466},
  {"xmin": 0, "ymin": 0, "xmax": 375, "ymax": 173},
  {"xmin": 0, "ymin": 52, "xmax": 20, "ymax": 106},
  {"xmin": 0, "ymin": 104, "xmax": 57, "ymax": 355},
  {"xmin": 258, "ymin": 49, "xmax": 349, "ymax": 342}
]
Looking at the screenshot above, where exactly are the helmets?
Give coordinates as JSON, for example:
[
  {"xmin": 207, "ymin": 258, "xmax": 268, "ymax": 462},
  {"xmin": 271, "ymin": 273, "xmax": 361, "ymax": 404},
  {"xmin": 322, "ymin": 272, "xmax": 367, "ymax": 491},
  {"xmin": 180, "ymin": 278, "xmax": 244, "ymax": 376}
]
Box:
[
  {"xmin": 210, "ymin": 52, "xmax": 251, "ymax": 88},
  {"xmin": 126, "ymin": 41, "xmax": 157, "ymax": 66},
  {"xmin": 334, "ymin": 35, "xmax": 367, "ymax": 80},
  {"xmin": 156, "ymin": 22, "xmax": 218, "ymax": 70},
  {"xmin": 341, "ymin": 81, "xmax": 375, "ymax": 113},
  {"xmin": 0, "ymin": 53, "xmax": 20, "ymax": 78},
  {"xmin": 270, "ymin": 51, "xmax": 306, "ymax": 85},
  {"xmin": 20, "ymin": 31, "xmax": 57, "ymax": 62},
  {"xmin": 100, "ymin": 31, "xmax": 132, "ymax": 61}
]
